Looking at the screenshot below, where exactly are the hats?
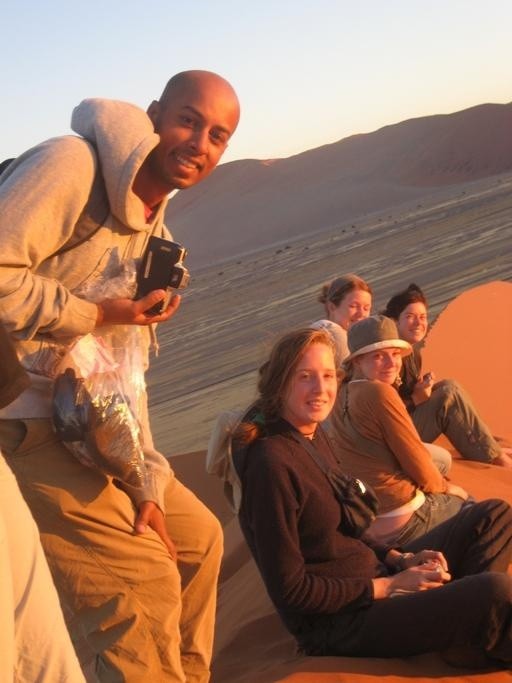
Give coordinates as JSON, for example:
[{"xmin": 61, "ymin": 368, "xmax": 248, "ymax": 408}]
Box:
[
  {"xmin": 311, "ymin": 321, "xmax": 350, "ymax": 368},
  {"xmin": 205, "ymin": 399, "xmax": 263, "ymax": 514},
  {"xmin": 343, "ymin": 315, "xmax": 412, "ymax": 366}
]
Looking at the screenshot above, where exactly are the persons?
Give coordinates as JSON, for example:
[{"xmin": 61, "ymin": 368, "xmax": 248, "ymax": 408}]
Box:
[
  {"xmin": 1, "ymin": 455, "xmax": 90, "ymax": 683},
  {"xmin": 382, "ymin": 282, "xmax": 512, "ymax": 469},
  {"xmin": 308, "ymin": 273, "xmax": 373, "ymax": 368},
  {"xmin": 0, "ymin": 69, "xmax": 240, "ymax": 683},
  {"xmin": 210, "ymin": 324, "xmax": 512, "ymax": 669},
  {"xmin": 327, "ymin": 315, "xmax": 469, "ymax": 550}
]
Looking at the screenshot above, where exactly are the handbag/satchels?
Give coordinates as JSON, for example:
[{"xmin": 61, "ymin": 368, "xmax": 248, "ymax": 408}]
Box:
[{"xmin": 336, "ymin": 477, "xmax": 380, "ymax": 539}]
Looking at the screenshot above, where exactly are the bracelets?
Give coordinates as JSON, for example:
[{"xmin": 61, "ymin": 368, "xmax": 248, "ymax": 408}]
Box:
[{"xmin": 396, "ymin": 552, "xmax": 414, "ymax": 570}]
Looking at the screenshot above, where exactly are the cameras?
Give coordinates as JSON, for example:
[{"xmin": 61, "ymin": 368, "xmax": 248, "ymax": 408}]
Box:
[
  {"xmin": 134, "ymin": 234, "xmax": 189, "ymax": 315},
  {"xmin": 423, "ymin": 374, "xmax": 431, "ymax": 387},
  {"xmin": 422, "ymin": 558, "xmax": 446, "ymax": 583}
]
